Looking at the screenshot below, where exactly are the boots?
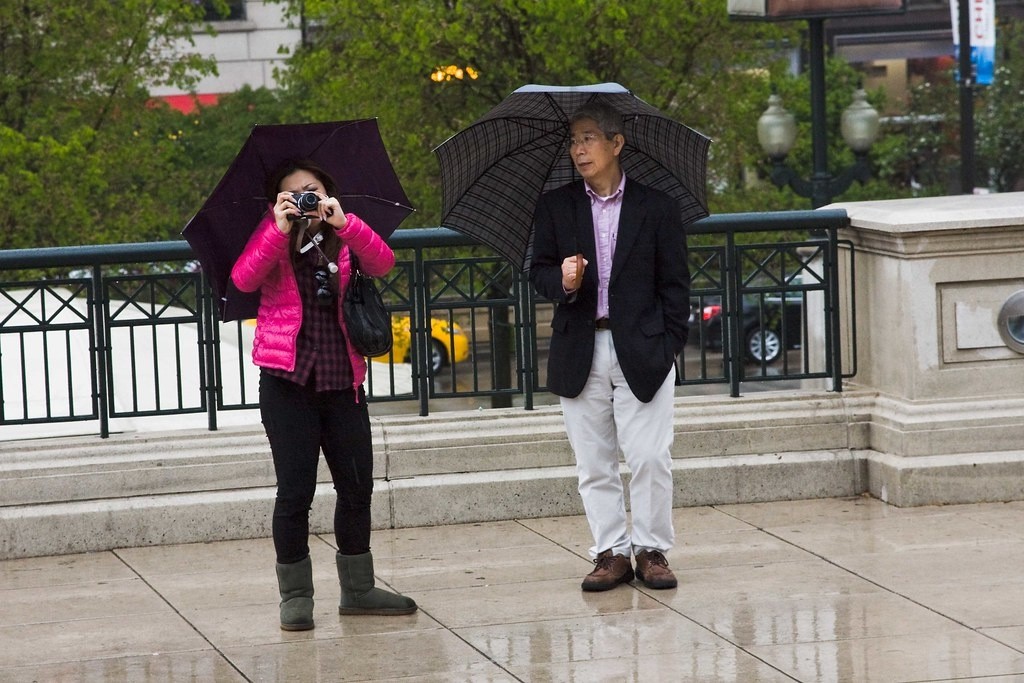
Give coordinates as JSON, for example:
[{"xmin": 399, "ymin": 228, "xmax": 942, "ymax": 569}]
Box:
[
  {"xmin": 276, "ymin": 555, "xmax": 315, "ymax": 631},
  {"xmin": 335, "ymin": 550, "xmax": 418, "ymax": 615}
]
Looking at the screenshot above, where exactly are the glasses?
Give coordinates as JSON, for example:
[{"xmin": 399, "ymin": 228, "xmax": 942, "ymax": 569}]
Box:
[{"xmin": 569, "ymin": 131, "xmax": 610, "ymax": 145}]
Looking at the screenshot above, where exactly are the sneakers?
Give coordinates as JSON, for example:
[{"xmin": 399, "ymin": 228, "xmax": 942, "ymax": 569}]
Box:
[
  {"xmin": 581, "ymin": 549, "xmax": 635, "ymax": 591},
  {"xmin": 635, "ymin": 549, "xmax": 678, "ymax": 589}
]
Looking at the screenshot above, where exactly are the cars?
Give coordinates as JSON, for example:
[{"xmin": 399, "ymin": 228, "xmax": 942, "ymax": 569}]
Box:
[
  {"xmin": 678, "ymin": 263, "xmax": 810, "ymax": 360},
  {"xmin": 371, "ymin": 314, "xmax": 472, "ymax": 376}
]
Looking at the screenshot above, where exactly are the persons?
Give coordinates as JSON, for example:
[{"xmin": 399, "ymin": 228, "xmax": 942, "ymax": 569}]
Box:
[
  {"xmin": 230, "ymin": 161, "xmax": 418, "ymax": 632},
  {"xmin": 530, "ymin": 103, "xmax": 691, "ymax": 591}
]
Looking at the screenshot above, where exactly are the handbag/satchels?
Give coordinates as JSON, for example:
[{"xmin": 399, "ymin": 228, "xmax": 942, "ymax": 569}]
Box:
[{"xmin": 342, "ymin": 249, "xmax": 394, "ymax": 357}]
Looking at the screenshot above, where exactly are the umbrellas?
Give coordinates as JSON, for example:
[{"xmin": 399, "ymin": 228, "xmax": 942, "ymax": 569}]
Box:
[
  {"xmin": 431, "ymin": 82, "xmax": 713, "ymax": 292},
  {"xmin": 180, "ymin": 117, "xmax": 416, "ymax": 324}
]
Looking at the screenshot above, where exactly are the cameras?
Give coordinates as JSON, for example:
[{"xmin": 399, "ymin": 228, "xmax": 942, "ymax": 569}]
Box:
[{"xmin": 286, "ymin": 192, "xmax": 323, "ymax": 220}]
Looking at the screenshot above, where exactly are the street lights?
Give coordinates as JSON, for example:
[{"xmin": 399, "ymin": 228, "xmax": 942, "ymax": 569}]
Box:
[{"xmin": 750, "ymin": 89, "xmax": 882, "ymax": 209}]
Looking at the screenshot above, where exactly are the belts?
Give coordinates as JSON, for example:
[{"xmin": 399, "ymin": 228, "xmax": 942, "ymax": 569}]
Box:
[{"xmin": 595, "ymin": 317, "xmax": 609, "ymax": 329}]
